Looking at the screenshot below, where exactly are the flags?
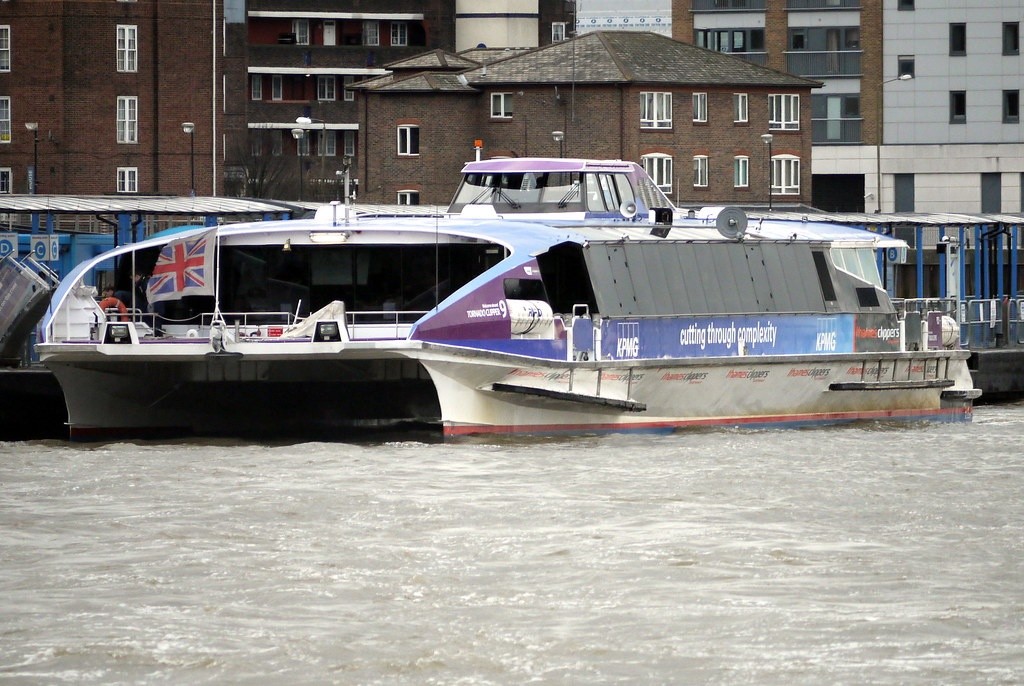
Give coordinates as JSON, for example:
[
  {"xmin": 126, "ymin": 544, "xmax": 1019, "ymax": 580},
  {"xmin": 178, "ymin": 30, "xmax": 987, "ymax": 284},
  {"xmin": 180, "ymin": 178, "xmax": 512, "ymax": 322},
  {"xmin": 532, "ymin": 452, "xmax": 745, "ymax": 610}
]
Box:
[{"xmin": 144, "ymin": 228, "xmax": 219, "ymax": 306}]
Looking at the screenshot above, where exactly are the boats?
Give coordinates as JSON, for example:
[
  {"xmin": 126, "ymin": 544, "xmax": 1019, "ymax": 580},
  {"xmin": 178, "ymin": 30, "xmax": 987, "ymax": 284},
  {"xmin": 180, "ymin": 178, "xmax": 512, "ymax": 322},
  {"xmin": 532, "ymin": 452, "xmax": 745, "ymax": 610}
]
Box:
[
  {"xmin": 400, "ymin": 341, "xmax": 972, "ymax": 437},
  {"xmin": 32, "ymin": 159, "xmax": 898, "ymax": 442}
]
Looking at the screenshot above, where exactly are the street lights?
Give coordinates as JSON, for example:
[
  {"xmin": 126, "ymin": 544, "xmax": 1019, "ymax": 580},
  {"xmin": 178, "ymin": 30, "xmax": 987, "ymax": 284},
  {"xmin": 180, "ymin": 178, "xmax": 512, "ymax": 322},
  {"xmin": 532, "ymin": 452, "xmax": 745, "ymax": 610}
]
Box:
[
  {"xmin": 760, "ymin": 133, "xmax": 773, "ymax": 211},
  {"xmin": 291, "ymin": 128, "xmax": 304, "ymax": 200},
  {"xmin": 876, "ymin": 74, "xmax": 913, "ymax": 212},
  {"xmin": 551, "ymin": 131, "xmax": 564, "ymax": 158},
  {"xmin": 296, "ymin": 116, "xmax": 326, "ymax": 200},
  {"xmin": 24, "ymin": 121, "xmax": 39, "ymax": 193},
  {"xmin": 181, "ymin": 122, "xmax": 196, "ymax": 196}
]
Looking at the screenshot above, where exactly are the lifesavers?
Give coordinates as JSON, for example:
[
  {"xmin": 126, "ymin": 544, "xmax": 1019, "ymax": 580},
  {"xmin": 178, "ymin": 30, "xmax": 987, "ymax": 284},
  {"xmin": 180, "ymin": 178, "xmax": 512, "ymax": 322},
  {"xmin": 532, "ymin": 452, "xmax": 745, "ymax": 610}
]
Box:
[{"xmin": 99, "ymin": 296, "xmax": 129, "ymax": 322}]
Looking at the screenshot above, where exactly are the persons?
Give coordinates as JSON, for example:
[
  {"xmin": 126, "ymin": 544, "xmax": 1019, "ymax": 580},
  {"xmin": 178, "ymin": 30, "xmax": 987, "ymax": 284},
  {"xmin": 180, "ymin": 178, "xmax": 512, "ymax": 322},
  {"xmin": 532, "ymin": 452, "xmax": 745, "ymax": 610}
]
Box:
[{"xmin": 103, "ymin": 270, "xmax": 164, "ymax": 338}]
